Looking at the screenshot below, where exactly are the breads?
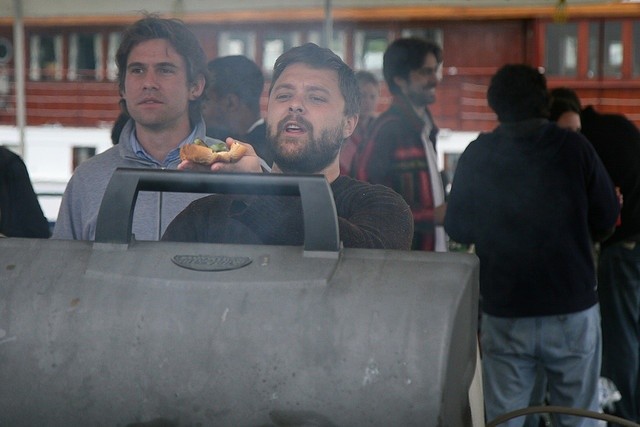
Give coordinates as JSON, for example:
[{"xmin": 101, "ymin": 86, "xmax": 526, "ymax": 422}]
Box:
[{"xmin": 176, "ymin": 137, "xmax": 249, "ymax": 167}]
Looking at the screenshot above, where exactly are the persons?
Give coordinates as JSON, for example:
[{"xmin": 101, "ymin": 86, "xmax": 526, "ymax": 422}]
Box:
[
  {"xmin": 202, "ymin": 55, "xmax": 267, "ymax": 149},
  {"xmin": 338, "ymin": 70, "xmax": 379, "ymax": 175},
  {"xmin": 160, "ymin": 43, "xmax": 415, "ymax": 250},
  {"xmin": 583, "ymin": 103, "xmax": 640, "ymax": 426},
  {"xmin": 50, "ymin": 9, "xmax": 271, "ymax": 240},
  {"xmin": 350, "ymin": 38, "xmax": 448, "ymax": 251},
  {"xmin": 546, "ymin": 87, "xmax": 582, "ymax": 131},
  {"xmin": 443, "ymin": 62, "xmax": 619, "ymax": 426}
]
